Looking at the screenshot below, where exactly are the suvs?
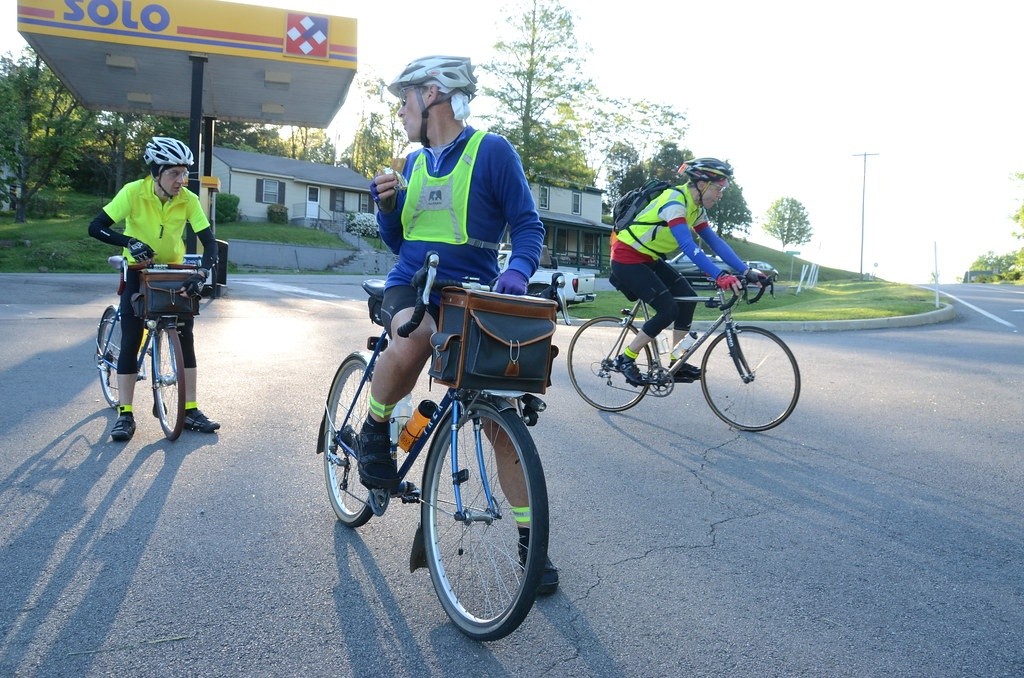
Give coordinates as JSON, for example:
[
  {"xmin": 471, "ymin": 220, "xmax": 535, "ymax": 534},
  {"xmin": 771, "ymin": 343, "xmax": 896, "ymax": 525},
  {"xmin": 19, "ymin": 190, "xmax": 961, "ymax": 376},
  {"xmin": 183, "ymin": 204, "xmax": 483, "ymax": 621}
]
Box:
[{"xmin": 664, "ymin": 250, "xmax": 740, "ymax": 290}]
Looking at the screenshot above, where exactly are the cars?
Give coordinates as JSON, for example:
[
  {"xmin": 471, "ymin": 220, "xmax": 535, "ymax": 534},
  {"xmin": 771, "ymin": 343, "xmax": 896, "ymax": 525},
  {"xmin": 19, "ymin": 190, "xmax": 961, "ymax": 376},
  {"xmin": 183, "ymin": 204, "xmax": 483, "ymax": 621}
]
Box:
[{"xmin": 743, "ymin": 260, "xmax": 781, "ymax": 281}]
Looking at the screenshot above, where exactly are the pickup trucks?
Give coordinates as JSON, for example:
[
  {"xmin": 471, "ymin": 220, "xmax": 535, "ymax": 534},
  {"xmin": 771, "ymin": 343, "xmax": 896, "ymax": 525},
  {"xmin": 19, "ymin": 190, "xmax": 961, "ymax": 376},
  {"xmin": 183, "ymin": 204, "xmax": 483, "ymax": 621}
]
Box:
[{"xmin": 490, "ymin": 244, "xmax": 598, "ymax": 311}]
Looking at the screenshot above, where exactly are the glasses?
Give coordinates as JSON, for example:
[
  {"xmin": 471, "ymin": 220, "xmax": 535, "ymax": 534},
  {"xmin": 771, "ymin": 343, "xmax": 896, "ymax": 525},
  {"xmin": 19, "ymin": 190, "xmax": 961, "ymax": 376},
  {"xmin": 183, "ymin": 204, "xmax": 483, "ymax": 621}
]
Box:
[
  {"xmin": 706, "ymin": 181, "xmax": 727, "ymax": 193},
  {"xmin": 161, "ymin": 168, "xmax": 189, "ymax": 178},
  {"xmin": 399, "ymin": 85, "xmax": 428, "ymax": 107}
]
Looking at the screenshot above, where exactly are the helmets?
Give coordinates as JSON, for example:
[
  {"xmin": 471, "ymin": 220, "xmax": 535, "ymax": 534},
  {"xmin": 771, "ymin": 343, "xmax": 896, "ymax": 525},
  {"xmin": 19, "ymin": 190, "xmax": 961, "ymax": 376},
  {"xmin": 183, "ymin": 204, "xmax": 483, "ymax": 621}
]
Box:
[
  {"xmin": 678, "ymin": 157, "xmax": 734, "ymax": 183},
  {"xmin": 143, "ymin": 137, "xmax": 195, "ymax": 181},
  {"xmin": 387, "ymin": 51, "xmax": 480, "ymax": 104}
]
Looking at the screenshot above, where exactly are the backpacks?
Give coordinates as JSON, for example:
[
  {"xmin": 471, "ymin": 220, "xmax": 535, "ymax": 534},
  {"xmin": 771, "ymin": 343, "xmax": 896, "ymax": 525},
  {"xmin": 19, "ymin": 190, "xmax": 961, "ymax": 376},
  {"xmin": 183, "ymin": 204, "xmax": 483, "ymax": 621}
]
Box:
[{"xmin": 612, "ymin": 178, "xmax": 703, "ymax": 261}]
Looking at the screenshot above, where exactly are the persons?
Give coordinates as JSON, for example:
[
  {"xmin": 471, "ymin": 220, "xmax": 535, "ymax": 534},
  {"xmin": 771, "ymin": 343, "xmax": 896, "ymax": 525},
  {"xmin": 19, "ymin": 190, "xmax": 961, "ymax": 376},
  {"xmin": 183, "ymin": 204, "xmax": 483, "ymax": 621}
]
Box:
[
  {"xmin": 610, "ymin": 158, "xmax": 769, "ymax": 386},
  {"xmin": 358, "ymin": 56, "xmax": 559, "ymax": 591},
  {"xmin": 88, "ymin": 137, "xmax": 221, "ymax": 441}
]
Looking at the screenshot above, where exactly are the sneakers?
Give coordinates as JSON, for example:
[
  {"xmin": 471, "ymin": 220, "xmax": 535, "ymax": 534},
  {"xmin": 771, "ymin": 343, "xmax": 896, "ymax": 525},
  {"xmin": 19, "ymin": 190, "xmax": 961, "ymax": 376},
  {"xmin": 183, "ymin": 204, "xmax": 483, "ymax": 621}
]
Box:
[
  {"xmin": 184, "ymin": 408, "xmax": 220, "ymax": 434},
  {"xmin": 111, "ymin": 415, "xmax": 136, "ymax": 441},
  {"xmin": 670, "ymin": 359, "xmax": 705, "ymax": 379},
  {"xmin": 615, "ymin": 352, "xmax": 646, "ymax": 386},
  {"xmin": 517, "ymin": 540, "xmax": 560, "ymax": 594},
  {"xmin": 353, "ymin": 425, "xmax": 401, "ymax": 490}
]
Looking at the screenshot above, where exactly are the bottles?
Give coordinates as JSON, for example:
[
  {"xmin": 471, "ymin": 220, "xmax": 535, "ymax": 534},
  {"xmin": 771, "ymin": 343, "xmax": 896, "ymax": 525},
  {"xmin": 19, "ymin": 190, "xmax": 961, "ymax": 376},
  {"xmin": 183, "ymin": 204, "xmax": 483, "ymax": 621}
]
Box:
[
  {"xmin": 671, "ymin": 330, "xmax": 698, "ymax": 359},
  {"xmin": 389, "ymin": 393, "xmax": 412, "ymax": 444},
  {"xmin": 397, "ymin": 400, "xmax": 438, "ymax": 451},
  {"xmin": 655, "ymin": 329, "xmax": 670, "ymax": 358},
  {"xmin": 136, "ymin": 324, "xmax": 149, "ymax": 354}
]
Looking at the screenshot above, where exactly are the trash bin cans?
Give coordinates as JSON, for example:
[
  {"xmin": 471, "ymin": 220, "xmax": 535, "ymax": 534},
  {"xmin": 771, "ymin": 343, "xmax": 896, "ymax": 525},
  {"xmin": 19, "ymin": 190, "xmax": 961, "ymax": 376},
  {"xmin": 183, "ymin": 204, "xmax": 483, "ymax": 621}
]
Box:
[{"xmin": 215, "ymin": 239, "xmax": 229, "ymax": 285}]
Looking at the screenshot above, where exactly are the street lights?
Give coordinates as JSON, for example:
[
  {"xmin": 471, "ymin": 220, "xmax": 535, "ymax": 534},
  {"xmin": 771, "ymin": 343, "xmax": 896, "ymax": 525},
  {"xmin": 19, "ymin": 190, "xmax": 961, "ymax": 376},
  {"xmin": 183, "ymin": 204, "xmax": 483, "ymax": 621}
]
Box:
[{"xmin": 853, "ymin": 152, "xmax": 878, "ymax": 280}]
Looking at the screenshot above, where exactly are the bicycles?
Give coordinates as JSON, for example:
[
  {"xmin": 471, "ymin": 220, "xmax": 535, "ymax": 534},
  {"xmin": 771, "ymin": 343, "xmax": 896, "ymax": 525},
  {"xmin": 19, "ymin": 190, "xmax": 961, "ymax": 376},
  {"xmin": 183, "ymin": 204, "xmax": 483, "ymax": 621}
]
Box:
[
  {"xmin": 92, "ymin": 256, "xmax": 226, "ymax": 442},
  {"xmin": 317, "ymin": 250, "xmax": 573, "ymax": 641},
  {"xmin": 566, "ymin": 272, "xmax": 802, "ymax": 432}
]
